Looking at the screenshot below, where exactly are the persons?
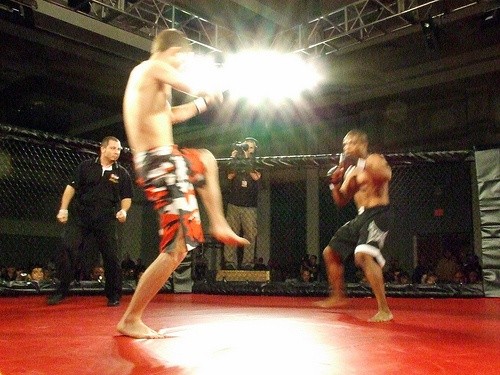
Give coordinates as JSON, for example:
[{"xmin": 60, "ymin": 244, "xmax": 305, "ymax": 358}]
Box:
[
  {"xmin": 224, "ymin": 138, "xmax": 262, "ymax": 270},
  {"xmin": 319, "ymin": 129, "xmax": 393, "ymax": 322},
  {"xmin": 121, "ymin": 253, "xmax": 146, "ymax": 280},
  {"xmin": 0, "ymin": 262, "xmax": 55, "ymax": 281},
  {"xmin": 253, "ymin": 253, "xmax": 320, "ymax": 282},
  {"xmin": 384, "ymin": 247, "xmax": 482, "ymax": 284},
  {"xmin": 47, "ymin": 135, "xmax": 132, "ymax": 306},
  {"xmin": 72, "ymin": 256, "xmax": 104, "ymax": 281},
  {"xmin": 115, "ymin": 28, "xmax": 250, "ymax": 339}
]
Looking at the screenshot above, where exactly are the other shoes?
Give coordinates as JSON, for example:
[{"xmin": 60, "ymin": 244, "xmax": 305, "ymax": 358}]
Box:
[
  {"xmin": 46, "ymin": 283, "xmax": 70, "ymax": 305},
  {"xmin": 107, "ymin": 298, "xmax": 120, "ymax": 306}
]
